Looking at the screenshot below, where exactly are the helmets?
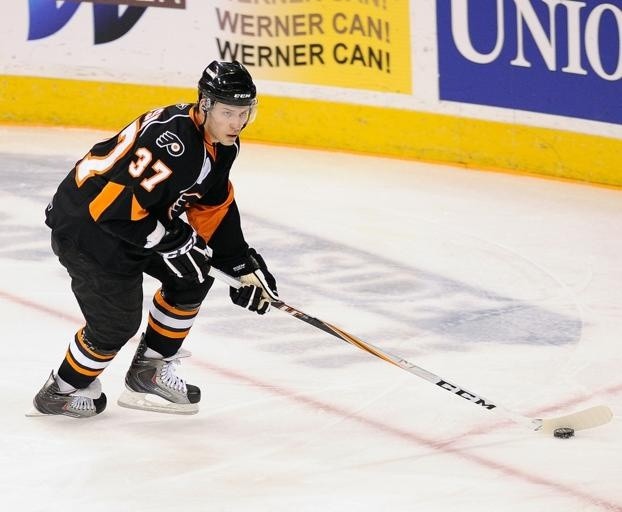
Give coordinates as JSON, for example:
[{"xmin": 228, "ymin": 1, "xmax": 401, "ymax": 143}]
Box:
[{"xmin": 196, "ymin": 60, "xmax": 259, "ymax": 123}]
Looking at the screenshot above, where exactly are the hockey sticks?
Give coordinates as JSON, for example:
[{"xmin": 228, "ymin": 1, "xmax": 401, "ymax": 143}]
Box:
[{"xmin": 209, "ymin": 267, "xmax": 612, "ymax": 432}]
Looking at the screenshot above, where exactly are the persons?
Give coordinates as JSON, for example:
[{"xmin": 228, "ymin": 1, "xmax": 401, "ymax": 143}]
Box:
[{"xmin": 30, "ymin": 57, "xmax": 280, "ymax": 417}]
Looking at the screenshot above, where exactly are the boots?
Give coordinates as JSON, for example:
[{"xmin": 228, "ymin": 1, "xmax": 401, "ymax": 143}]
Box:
[
  {"xmin": 117, "ymin": 332, "xmax": 201, "ymax": 415},
  {"xmin": 33, "ymin": 369, "xmax": 108, "ymax": 419}
]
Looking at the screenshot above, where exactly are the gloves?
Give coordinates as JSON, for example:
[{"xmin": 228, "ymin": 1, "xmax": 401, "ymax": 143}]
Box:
[
  {"xmin": 229, "ymin": 246, "xmax": 279, "ymax": 315},
  {"xmin": 154, "ymin": 217, "xmax": 213, "ymax": 286}
]
